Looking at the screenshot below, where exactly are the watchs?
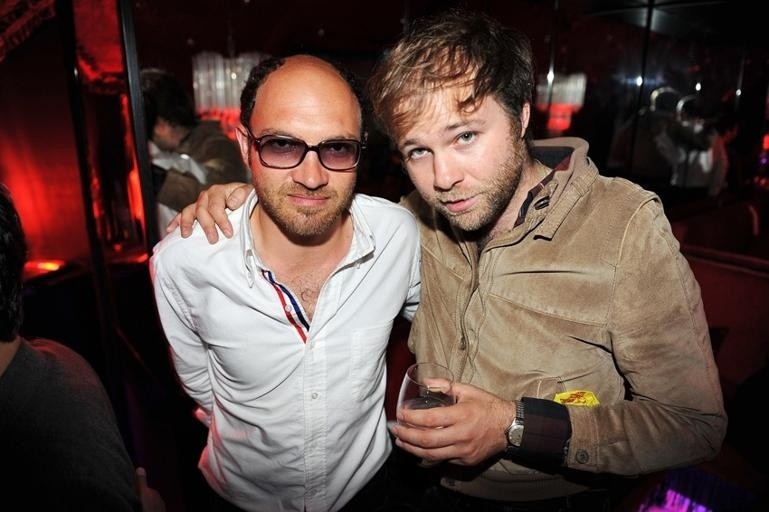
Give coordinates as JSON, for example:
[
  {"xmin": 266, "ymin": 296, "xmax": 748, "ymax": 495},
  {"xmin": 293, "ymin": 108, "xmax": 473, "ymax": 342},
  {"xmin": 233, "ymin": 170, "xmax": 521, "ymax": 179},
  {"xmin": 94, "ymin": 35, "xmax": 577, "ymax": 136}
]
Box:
[{"xmin": 504, "ymin": 400, "xmax": 526, "ymax": 455}]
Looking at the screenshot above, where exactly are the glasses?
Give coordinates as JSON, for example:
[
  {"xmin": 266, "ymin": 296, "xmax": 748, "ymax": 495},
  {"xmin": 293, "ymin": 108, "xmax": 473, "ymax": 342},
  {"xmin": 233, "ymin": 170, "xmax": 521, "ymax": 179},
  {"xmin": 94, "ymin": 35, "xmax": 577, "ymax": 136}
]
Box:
[{"xmin": 247, "ymin": 122, "xmax": 367, "ymax": 174}]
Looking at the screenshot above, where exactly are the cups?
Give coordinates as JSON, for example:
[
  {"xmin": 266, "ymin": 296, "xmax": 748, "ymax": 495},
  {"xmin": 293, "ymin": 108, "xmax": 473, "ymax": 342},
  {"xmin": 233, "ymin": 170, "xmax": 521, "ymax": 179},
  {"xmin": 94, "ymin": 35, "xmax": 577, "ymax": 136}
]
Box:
[{"xmin": 395, "ymin": 361, "xmax": 459, "ymax": 431}]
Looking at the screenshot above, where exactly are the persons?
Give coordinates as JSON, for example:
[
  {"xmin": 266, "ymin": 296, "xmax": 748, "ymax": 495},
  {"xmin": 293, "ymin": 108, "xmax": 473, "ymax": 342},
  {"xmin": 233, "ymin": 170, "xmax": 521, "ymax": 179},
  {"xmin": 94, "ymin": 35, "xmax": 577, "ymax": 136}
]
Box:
[
  {"xmin": 661, "ymin": 93, "xmax": 732, "ymax": 210},
  {"xmin": 609, "ymin": 88, "xmax": 719, "ymax": 187},
  {"xmin": 147, "ymin": 80, "xmax": 248, "ymax": 239},
  {"xmin": 1, "ymin": 184, "xmax": 165, "ymax": 511},
  {"xmin": 149, "ymin": 52, "xmax": 425, "ymax": 512},
  {"xmin": 163, "ymin": 14, "xmax": 731, "ymax": 512}
]
